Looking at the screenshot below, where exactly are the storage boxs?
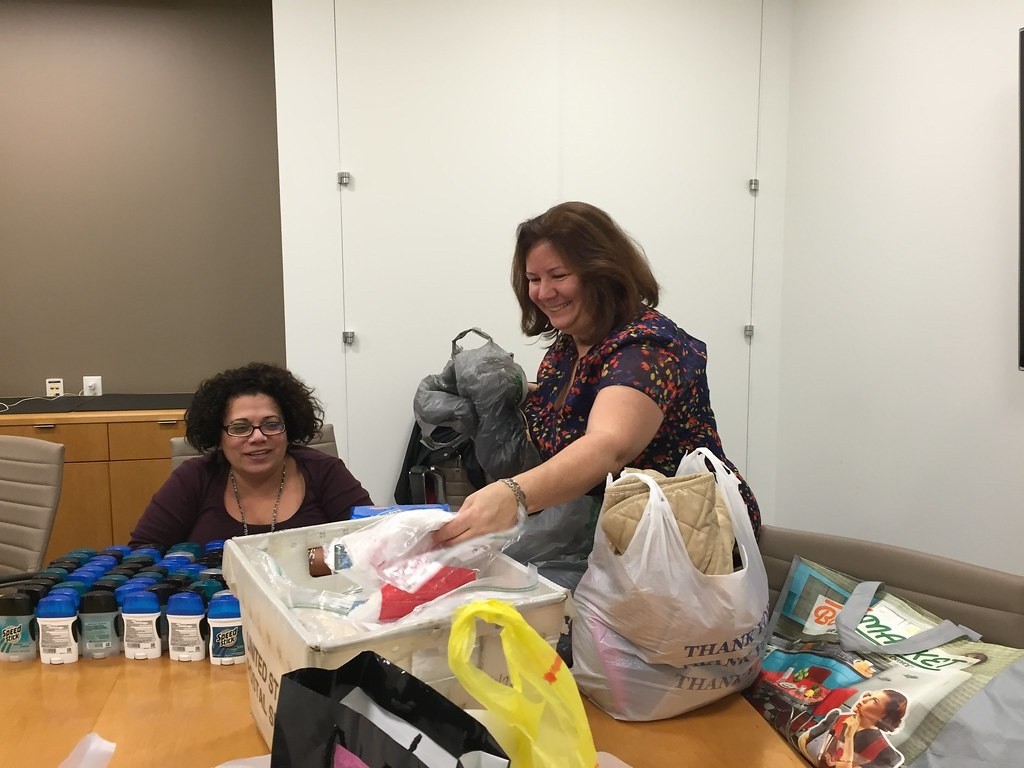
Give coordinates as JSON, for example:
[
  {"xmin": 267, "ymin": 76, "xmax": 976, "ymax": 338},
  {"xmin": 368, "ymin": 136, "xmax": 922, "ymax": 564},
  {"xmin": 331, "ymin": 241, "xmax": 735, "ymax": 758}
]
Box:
[{"xmin": 222, "ymin": 507, "xmax": 568, "ymax": 755}]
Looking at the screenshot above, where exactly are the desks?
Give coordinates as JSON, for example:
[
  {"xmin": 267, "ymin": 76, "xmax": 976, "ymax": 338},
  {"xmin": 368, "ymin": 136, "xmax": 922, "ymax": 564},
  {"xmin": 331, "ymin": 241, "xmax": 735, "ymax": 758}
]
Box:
[{"xmin": 0, "ymin": 659, "xmax": 813, "ymax": 768}]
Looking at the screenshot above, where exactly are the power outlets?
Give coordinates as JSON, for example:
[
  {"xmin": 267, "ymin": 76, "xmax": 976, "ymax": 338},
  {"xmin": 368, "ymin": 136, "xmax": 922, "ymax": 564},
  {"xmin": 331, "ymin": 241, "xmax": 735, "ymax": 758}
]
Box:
[
  {"xmin": 83, "ymin": 376, "xmax": 102, "ymax": 396},
  {"xmin": 46, "ymin": 379, "xmax": 64, "ymax": 397}
]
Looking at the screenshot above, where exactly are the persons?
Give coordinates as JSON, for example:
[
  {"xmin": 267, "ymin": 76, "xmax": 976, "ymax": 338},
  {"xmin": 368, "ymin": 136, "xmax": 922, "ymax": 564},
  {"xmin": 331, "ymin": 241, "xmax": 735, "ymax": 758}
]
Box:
[
  {"xmin": 126, "ymin": 362, "xmax": 375, "ymax": 552},
  {"xmin": 431, "ymin": 200, "xmax": 762, "ymax": 574},
  {"xmin": 798, "ymin": 689, "xmax": 908, "ymax": 768}
]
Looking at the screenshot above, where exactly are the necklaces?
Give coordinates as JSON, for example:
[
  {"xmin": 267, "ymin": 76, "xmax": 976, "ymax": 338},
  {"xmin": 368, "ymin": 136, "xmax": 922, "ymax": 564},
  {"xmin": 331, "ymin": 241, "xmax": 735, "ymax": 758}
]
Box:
[{"xmin": 229, "ymin": 459, "xmax": 286, "ymax": 536}]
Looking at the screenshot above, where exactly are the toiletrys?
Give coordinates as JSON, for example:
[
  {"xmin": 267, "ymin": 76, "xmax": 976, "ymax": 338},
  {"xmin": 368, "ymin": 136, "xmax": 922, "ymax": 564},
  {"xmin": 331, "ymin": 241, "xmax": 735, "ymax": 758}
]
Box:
[{"xmin": 0, "ymin": 538, "xmax": 248, "ymax": 666}]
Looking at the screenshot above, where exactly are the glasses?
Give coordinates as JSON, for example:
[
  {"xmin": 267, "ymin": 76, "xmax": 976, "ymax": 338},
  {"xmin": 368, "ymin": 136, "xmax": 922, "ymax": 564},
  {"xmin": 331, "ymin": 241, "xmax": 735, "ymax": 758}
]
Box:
[{"xmin": 222, "ymin": 422, "xmax": 286, "ymax": 437}]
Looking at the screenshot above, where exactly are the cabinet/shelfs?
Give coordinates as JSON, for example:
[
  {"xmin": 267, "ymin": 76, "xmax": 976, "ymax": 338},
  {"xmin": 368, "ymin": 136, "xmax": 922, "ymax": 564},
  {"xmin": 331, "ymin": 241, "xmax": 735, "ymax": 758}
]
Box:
[{"xmin": 0, "ymin": 408, "xmax": 221, "ymax": 571}]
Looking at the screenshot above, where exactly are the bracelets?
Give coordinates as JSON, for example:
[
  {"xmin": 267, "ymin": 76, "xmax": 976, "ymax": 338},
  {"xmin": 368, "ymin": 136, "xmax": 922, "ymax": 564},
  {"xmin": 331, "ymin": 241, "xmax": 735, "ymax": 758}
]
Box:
[{"xmin": 496, "ymin": 478, "xmax": 528, "ymax": 524}]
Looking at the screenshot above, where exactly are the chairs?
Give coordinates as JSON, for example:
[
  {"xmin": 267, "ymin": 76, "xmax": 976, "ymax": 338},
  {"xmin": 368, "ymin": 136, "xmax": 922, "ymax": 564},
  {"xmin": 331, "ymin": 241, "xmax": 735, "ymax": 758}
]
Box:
[
  {"xmin": 0, "ymin": 433, "xmax": 64, "ymax": 577},
  {"xmin": 170, "ymin": 424, "xmax": 339, "ymax": 476}
]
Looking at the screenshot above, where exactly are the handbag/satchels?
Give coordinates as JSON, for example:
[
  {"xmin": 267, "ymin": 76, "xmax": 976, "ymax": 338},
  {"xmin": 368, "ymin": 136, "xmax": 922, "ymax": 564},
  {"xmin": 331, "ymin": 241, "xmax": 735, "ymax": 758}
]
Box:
[
  {"xmin": 741, "ymin": 555, "xmax": 1024, "ymax": 768},
  {"xmin": 570, "ymin": 447, "xmax": 769, "ymax": 721},
  {"xmin": 271, "ymin": 652, "xmax": 511, "ymax": 768},
  {"xmin": 413, "ymin": 327, "xmax": 542, "ymax": 480},
  {"xmin": 447, "ymin": 599, "xmax": 599, "ymax": 768}
]
